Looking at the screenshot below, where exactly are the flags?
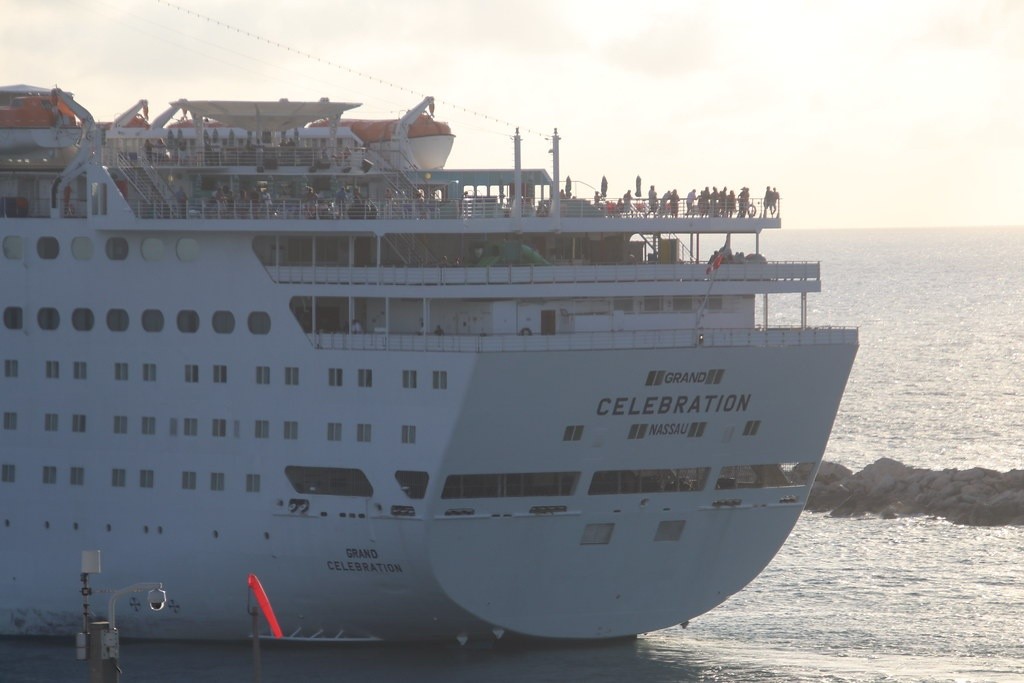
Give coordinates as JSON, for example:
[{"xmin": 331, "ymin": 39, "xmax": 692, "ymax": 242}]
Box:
[{"xmin": 706, "ymin": 252, "xmax": 724, "ymax": 274}]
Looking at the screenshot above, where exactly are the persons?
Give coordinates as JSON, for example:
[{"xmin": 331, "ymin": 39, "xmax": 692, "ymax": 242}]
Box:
[
  {"xmin": 343, "ymin": 145, "xmax": 352, "ymax": 166},
  {"xmin": 439, "ymin": 256, "xmax": 452, "ymax": 267},
  {"xmin": 145, "ymin": 138, "xmax": 168, "ymax": 165},
  {"xmin": 174, "ymin": 185, "xmax": 427, "ymax": 220},
  {"xmin": 280, "ymin": 138, "xmax": 296, "ymax": 165},
  {"xmin": 615, "ymin": 185, "xmax": 779, "ymax": 218}
]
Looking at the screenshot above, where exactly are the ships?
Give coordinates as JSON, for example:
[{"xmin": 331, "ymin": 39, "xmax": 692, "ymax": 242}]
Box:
[{"xmin": 0, "ymin": 83, "xmax": 860, "ymax": 644}]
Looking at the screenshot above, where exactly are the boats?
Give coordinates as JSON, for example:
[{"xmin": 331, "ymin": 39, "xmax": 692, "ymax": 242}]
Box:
[
  {"xmin": 0, "ymin": 83, "xmax": 84, "ymax": 151},
  {"xmin": 308, "ymin": 109, "xmax": 455, "ymax": 169}
]
